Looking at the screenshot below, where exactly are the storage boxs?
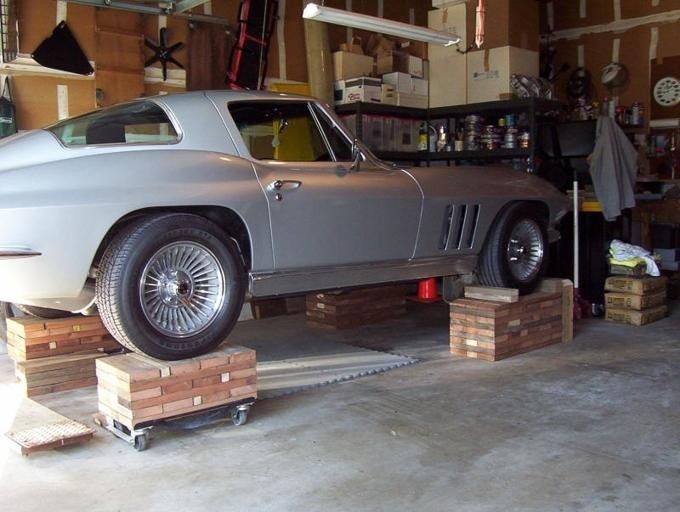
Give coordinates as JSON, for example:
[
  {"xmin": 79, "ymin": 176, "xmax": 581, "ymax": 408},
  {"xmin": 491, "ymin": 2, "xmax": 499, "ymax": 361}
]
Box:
[{"xmin": 333, "ymin": 0, "xmax": 541, "ymax": 152}]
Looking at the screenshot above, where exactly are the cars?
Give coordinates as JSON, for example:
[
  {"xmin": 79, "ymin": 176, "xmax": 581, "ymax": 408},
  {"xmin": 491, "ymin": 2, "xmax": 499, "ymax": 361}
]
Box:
[{"xmin": 0, "ymin": 88, "xmax": 572, "ymax": 361}]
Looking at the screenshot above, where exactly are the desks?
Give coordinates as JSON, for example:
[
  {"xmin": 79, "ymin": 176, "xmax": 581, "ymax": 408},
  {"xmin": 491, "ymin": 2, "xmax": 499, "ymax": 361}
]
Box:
[
  {"xmin": 631, "ymin": 199, "xmax": 680, "ymax": 255},
  {"xmin": 557, "ymin": 202, "xmax": 631, "ymax": 277}
]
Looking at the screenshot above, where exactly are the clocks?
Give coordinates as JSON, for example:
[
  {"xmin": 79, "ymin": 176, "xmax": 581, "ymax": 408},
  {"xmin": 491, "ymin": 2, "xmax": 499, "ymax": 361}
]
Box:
[{"xmin": 653, "ymin": 76, "xmax": 680, "ymax": 107}]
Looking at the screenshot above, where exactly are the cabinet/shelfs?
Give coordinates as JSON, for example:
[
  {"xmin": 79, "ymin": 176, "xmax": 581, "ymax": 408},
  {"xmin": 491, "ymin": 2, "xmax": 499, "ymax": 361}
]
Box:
[{"xmin": 335, "ymin": 98, "xmax": 563, "ymax": 173}]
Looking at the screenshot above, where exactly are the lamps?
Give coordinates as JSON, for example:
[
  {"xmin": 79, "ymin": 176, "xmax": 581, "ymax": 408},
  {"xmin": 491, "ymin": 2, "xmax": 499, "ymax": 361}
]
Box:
[{"xmin": 302, "ymin": 0, "xmax": 462, "ymax": 47}]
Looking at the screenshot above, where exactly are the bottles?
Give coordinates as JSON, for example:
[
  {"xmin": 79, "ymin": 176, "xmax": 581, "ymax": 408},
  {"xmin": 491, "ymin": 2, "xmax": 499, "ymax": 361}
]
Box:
[
  {"xmin": 602, "ymin": 97, "xmax": 615, "ymax": 119},
  {"xmin": 632, "ymin": 101, "xmax": 640, "ymax": 125}
]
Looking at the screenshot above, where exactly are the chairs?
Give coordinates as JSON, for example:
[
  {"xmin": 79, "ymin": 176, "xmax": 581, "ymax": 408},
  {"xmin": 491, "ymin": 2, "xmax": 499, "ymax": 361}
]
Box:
[{"xmin": 85, "ymin": 123, "xmax": 126, "ymax": 143}]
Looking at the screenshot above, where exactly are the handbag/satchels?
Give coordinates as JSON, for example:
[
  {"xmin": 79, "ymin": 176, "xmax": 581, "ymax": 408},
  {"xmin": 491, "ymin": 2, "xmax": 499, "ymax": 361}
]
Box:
[
  {"xmin": 31, "ymin": 21, "xmax": 94, "ymax": 74},
  {"xmin": 0, "ymin": 97, "xmax": 16, "ymax": 138}
]
